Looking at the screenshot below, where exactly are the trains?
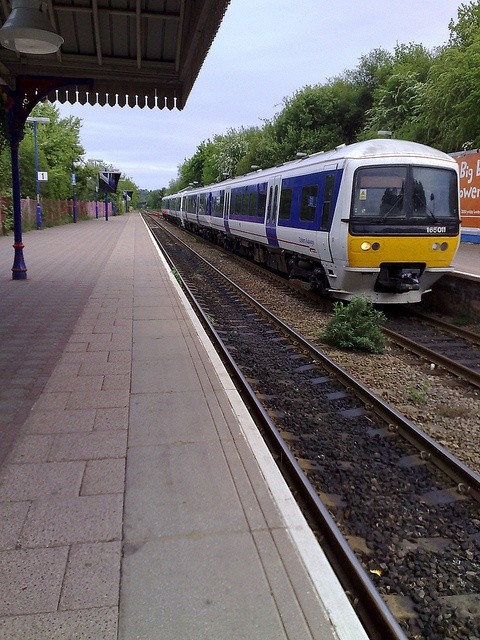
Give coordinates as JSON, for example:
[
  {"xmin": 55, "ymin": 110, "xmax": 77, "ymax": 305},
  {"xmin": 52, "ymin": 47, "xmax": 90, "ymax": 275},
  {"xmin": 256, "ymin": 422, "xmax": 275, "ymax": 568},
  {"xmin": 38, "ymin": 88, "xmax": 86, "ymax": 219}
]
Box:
[{"xmin": 161, "ymin": 138, "xmax": 462, "ymax": 305}]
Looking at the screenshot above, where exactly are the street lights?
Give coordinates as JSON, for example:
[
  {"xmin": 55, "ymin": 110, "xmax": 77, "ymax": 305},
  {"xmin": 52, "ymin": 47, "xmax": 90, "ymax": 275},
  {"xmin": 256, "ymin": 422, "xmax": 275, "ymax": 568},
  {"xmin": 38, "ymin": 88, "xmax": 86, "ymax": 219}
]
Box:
[
  {"xmin": 94, "ymin": 185, "xmax": 100, "ymax": 218},
  {"xmin": 71, "ymin": 158, "xmax": 81, "ymax": 224},
  {"xmin": 26, "ymin": 116, "xmax": 51, "ymax": 228}
]
